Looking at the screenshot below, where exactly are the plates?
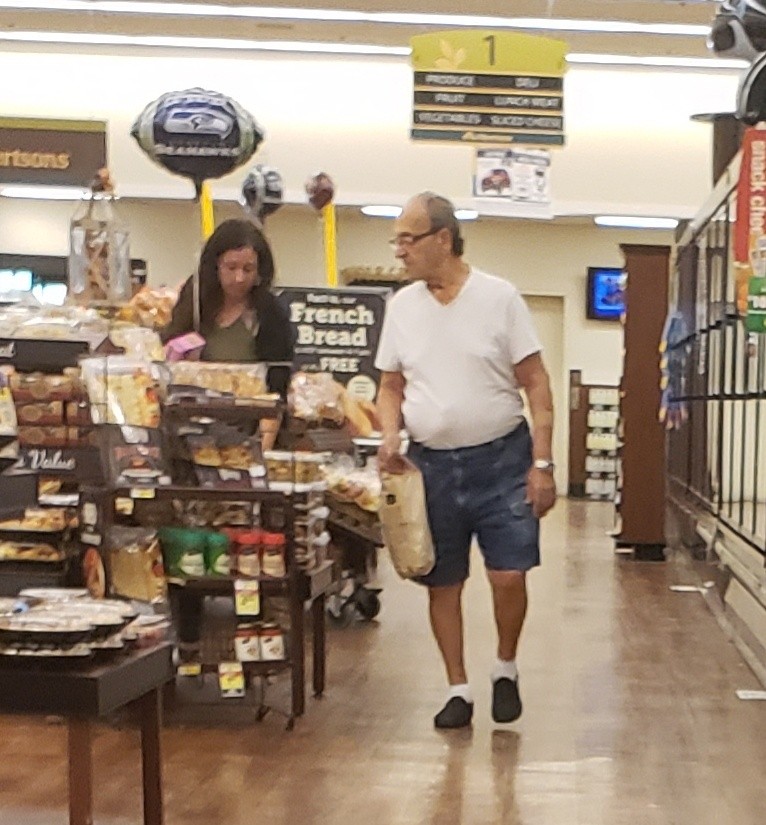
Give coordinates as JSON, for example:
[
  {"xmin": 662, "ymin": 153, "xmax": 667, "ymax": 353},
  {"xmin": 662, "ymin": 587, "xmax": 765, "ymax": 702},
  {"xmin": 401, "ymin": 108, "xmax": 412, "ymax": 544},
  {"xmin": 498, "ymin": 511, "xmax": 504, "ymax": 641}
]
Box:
[{"xmin": 0, "ymin": 586, "xmax": 142, "ymax": 669}]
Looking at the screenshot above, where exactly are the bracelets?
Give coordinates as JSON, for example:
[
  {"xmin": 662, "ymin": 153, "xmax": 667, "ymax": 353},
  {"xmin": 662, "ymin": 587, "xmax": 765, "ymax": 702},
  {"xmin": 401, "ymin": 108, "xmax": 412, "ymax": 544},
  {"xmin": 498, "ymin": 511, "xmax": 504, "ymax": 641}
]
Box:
[{"xmin": 534, "ymin": 459, "xmax": 555, "ymax": 470}]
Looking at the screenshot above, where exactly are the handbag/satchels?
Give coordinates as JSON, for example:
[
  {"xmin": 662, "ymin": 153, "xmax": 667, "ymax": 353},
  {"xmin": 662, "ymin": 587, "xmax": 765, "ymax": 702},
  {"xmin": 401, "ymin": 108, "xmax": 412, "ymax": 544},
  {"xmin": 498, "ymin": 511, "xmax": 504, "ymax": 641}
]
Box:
[{"xmin": 377, "ymin": 452, "xmax": 436, "ymax": 579}]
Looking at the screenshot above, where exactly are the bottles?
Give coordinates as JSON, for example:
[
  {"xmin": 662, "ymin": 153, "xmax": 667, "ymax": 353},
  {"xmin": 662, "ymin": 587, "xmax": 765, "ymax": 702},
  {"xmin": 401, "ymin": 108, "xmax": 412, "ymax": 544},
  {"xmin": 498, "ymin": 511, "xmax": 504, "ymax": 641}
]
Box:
[
  {"xmin": 158, "ymin": 524, "xmax": 289, "ymax": 581},
  {"xmin": 233, "ymin": 620, "xmax": 287, "ymax": 661}
]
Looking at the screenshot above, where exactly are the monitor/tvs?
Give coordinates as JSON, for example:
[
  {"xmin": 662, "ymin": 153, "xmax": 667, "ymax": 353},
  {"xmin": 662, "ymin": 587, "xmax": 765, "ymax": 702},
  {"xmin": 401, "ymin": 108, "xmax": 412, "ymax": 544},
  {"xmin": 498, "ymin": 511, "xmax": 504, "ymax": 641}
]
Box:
[{"xmin": 586, "ymin": 267, "xmax": 626, "ymax": 321}]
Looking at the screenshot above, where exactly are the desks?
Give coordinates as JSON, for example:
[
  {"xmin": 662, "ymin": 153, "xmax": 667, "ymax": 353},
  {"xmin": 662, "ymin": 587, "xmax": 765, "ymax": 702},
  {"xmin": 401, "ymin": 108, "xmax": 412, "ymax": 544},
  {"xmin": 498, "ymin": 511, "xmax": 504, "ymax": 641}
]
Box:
[
  {"xmin": 0, "ymin": 638, "xmax": 181, "ymax": 825},
  {"xmin": 103, "ymin": 479, "xmax": 333, "ymax": 730},
  {"xmin": 164, "ymin": 558, "xmax": 339, "ymax": 714}
]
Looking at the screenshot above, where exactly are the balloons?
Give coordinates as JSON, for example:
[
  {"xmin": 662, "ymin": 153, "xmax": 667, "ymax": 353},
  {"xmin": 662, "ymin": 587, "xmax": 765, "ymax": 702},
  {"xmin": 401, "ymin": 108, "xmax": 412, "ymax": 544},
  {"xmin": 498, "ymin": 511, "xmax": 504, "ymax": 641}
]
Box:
[
  {"xmin": 239, "ymin": 165, "xmax": 284, "ymax": 223},
  {"xmin": 131, "ymin": 88, "xmax": 263, "ymax": 204},
  {"xmin": 305, "ymin": 171, "xmax": 334, "ymax": 216}
]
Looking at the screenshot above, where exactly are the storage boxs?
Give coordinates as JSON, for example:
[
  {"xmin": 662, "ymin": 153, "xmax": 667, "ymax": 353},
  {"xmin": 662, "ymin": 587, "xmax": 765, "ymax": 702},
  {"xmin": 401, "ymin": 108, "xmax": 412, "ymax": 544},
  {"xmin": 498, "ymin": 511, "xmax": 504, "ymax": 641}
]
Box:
[
  {"xmin": 293, "ymin": 541, "xmax": 318, "ymax": 555},
  {"xmin": 165, "ymin": 330, "xmax": 207, "ymax": 363},
  {"xmin": 585, "ymin": 388, "xmax": 619, "ymax": 493},
  {"xmin": 7, "ymin": 367, "xmax": 96, "ymax": 447},
  {"xmin": 312, "ymin": 506, "xmax": 334, "ymax": 535},
  {"xmin": 217, "ymin": 661, "xmax": 246, "ymax": 699},
  {"xmin": 264, "ymin": 450, "xmax": 327, "ymax": 479},
  {"xmin": 315, "ymin": 531, "xmax": 335, "ymax": 563},
  {"xmin": 292, "ymin": 492, "xmax": 315, "ymax": 520},
  {"xmin": 296, "ymin": 516, "xmax": 316, "ymax": 542}
]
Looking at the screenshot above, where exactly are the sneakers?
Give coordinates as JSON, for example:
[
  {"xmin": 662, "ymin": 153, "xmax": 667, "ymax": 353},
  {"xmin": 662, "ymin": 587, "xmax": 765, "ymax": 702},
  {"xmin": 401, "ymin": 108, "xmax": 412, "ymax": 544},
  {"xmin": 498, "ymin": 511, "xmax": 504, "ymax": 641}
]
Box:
[
  {"xmin": 492, "ymin": 677, "xmax": 522, "ymax": 723},
  {"xmin": 434, "ymin": 697, "xmax": 473, "ymax": 728}
]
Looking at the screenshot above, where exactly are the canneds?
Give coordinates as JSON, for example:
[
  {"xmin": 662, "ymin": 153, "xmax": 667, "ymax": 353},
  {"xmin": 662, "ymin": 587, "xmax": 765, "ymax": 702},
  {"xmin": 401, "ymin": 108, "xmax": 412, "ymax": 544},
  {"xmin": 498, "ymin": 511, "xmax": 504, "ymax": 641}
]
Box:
[
  {"xmin": 234, "ymin": 621, "xmax": 289, "ymax": 661},
  {"xmin": 209, "ymin": 529, "xmax": 286, "ymax": 578}
]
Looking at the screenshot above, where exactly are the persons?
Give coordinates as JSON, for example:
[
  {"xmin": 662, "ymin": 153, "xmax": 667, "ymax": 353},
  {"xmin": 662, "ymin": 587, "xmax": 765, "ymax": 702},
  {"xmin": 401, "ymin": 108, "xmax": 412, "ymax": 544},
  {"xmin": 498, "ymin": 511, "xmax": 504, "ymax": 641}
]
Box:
[
  {"xmin": 373, "ymin": 191, "xmax": 558, "ymax": 729},
  {"xmin": 601, "ymin": 278, "xmax": 623, "ymax": 305},
  {"xmin": 169, "ymin": 221, "xmax": 299, "ymax": 665}
]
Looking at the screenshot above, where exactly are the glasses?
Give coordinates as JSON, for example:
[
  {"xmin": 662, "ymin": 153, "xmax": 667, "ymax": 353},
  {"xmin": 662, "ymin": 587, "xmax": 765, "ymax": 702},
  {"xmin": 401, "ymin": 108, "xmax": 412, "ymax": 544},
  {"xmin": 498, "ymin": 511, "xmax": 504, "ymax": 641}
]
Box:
[{"xmin": 387, "ymin": 224, "xmax": 443, "ymax": 250}]
